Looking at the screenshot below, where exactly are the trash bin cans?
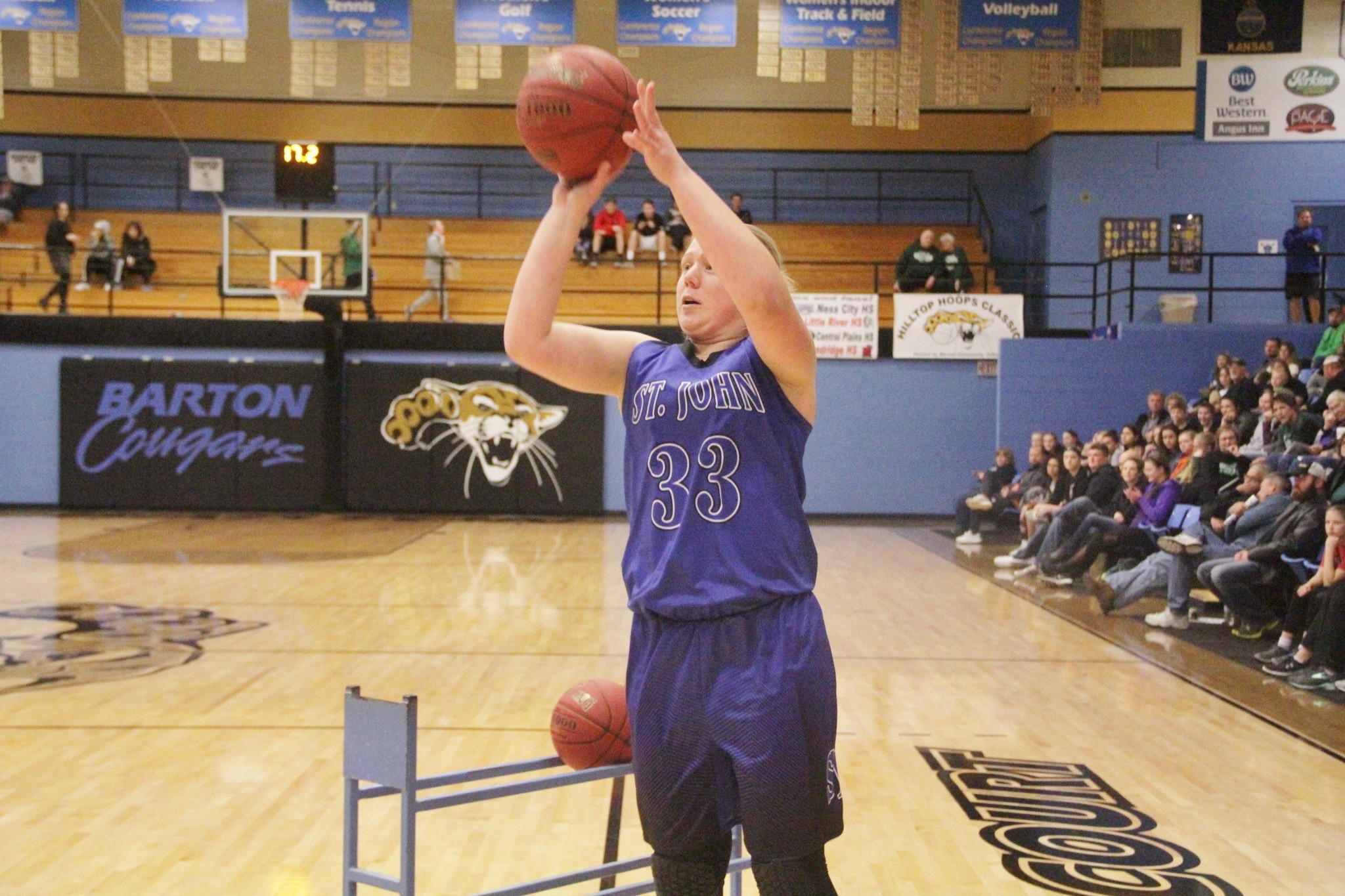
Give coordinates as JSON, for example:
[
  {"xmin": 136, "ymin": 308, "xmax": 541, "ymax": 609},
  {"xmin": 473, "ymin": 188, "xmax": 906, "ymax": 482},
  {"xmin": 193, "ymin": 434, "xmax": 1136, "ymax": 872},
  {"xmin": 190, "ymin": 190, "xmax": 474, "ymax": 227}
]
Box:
[{"xmin": 1159, "ymin": 294, "xmax": 1198, "ymax": 324}]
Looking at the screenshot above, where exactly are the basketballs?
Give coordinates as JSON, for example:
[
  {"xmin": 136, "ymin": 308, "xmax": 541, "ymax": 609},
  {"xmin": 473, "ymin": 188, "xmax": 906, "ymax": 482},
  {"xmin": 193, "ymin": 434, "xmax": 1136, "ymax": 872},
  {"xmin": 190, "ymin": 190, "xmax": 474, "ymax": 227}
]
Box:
[
  {"xmin": 515, "ymin": 44, "xmax": 639, "ymax": 184},
  {"xmin": 549, "ymin": 679, "xmax": 632, "ymax": 771}
]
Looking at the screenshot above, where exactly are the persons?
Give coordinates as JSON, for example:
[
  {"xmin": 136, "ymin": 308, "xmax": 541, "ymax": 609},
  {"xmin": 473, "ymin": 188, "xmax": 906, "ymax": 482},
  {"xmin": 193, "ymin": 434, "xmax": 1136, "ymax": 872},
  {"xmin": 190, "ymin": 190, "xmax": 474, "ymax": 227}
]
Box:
[
  {"xmin": 0, "ymin": 179, "xmax": 21, "ymax": 237},
  {"xmin": 1282, "ymin": 210, "xmax": 1323, "ymax": 324},
  {"xmin": 573, "ymin": 194, "xmax": 751, "ymax": 270},
  {"xmin": 945, "ymin": 304, "xmax": 1345, "ymax": 691},
  {"xmin": 893, "ymin": 230, "xmax": 974, "ymax": 294},
  {"xmin": 341, "ymin": 220, "xmax": 382, "ymax": 322},
  {"xmin": 39, "ymin": 202, "xmax": 78, "ymax": 316},
  {"xmin": 75, "ymin": 219, "xmax": 156, "ymax": 292},
  {"xmin": 402, "ymin": 220, "xmax": 457, "ymax": 323},
  {"xmin": 504, "ymin": 78, "xmax": 844, "ymax": 896}
]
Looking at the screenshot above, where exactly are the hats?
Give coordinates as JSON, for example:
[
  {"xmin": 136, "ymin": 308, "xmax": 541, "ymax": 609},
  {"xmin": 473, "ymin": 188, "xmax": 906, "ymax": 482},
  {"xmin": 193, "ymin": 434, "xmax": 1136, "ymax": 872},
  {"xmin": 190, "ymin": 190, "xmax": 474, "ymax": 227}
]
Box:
[{"xmin": 1285, "ymin": 460, "xmax": 1325, "ymax": 482}]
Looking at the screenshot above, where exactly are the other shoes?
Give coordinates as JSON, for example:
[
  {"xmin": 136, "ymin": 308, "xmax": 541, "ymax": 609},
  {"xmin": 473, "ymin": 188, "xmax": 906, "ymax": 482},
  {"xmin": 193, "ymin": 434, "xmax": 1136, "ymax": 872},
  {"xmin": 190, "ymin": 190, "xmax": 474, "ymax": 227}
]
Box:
[
  {"xmin": 952, "ymin": 527, "xmax": 1118, "ymax": 615},
  {"xmin": 1335, "ymin": 680, "xmax": 1345, "ymax": 692}
]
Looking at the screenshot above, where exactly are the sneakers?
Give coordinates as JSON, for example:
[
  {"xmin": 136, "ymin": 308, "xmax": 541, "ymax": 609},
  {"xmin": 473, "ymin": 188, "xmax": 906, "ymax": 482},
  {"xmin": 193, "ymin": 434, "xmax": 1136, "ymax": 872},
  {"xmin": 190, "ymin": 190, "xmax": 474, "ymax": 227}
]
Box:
[
  {"xmin": 1231, "ymin": 616, "xmax": 1279, "ymax": 638},
  {"xmin": 1145, "ymin": 607, "xmax": 1189, "ymax": 629},
  {"xmin": 966, "ymin": 494, "xmax": 993, "ymax": 511},
  {"xmin": 1254, "ymin": 646, "xmax": 1294, "ymax": 663},
  {"xmin": 1288, "ymin": 667, "xmax": 1341, "ymax": 689},
  {"xmin": 1157, "ymin": 536, "xmax": 1203, "ymax": 556},
  {"xmin": 1262, "ymin": 658, "xmax": 1305, "ymax": 676},
  {"xmin": 1270, "ymin": 654, "xmax": 1292, "ymax": 666}
]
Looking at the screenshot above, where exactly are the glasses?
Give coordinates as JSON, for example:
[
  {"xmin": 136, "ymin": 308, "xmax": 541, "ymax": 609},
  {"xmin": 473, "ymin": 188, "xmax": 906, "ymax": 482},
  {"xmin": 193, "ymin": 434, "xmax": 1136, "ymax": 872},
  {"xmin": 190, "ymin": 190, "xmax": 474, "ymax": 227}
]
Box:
[{"xmin": 1243, "ymin": 474, "xmax": 1261, "ymax": 484}]
